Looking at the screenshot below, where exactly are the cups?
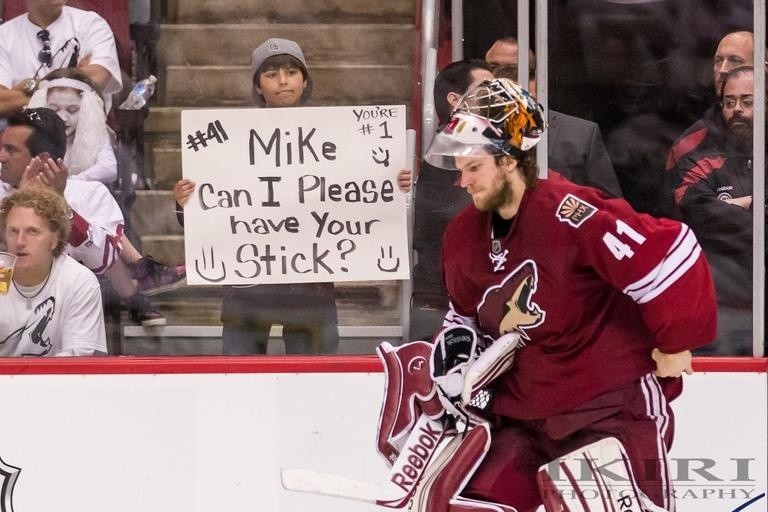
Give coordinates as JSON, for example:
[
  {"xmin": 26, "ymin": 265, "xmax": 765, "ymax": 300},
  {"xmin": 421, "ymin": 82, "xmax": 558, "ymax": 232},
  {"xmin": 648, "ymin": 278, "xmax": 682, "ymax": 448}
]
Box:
[{"xmin": 0, "ymin": 250, "xmax": 20, "ymax": 296}]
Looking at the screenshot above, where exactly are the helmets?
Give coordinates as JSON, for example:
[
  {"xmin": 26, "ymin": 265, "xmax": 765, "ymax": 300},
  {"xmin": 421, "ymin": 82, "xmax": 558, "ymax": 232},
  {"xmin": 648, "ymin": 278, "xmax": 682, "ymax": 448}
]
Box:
[{"xmin": 423, "ymin": 77, "xmax": 547, "ymax": 169}]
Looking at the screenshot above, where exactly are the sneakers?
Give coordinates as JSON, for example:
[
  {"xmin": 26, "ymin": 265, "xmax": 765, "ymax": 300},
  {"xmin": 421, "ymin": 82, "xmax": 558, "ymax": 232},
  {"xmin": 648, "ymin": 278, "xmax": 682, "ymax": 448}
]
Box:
[
  {"xmin": 129, "ymin": 255, "xmax": 187, "ymax": 298},
  {"xmin": 126, "ymin": 292, "xmax": 167, "ymax": 329}
]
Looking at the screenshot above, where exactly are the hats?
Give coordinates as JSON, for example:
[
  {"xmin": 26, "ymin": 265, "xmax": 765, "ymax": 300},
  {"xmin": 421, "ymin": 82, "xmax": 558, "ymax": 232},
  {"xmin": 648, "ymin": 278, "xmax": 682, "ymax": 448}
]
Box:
[{"xmin": 249, "ymin": 37, "xmax": 312, "ymax": 105}]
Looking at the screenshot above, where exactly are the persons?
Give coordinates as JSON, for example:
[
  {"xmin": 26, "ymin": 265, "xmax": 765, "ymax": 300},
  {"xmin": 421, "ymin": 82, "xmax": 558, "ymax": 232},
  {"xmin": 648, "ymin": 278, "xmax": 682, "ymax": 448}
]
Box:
[
  {"xmin": 173, "ymin": 36, "xmax": 411, "ymax": 356},
  {"xmin": 423, "ymin": 77, "xmax": 718, "ymax": 512},
  {"xmin": 0, "ymin": 0, "xmax": 186, "ymax": 356},
  {"xmin": 413, "ymin": 30, "xmax": 765, "ymax": 356}
]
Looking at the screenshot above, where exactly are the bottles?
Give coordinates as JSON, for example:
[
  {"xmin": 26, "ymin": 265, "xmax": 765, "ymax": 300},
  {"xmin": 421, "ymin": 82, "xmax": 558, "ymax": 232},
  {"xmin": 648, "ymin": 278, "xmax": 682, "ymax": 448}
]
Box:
[{"xmin": 117, "ymin": 72, "xmax": 158, "ymax": 111}]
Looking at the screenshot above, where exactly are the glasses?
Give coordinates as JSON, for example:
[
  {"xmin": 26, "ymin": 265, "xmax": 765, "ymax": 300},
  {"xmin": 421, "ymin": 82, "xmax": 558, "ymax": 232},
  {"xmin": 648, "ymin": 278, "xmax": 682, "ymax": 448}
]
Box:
[
  {"xmin": 37, "ymin": 30, "xmax": 53, "ymax": 67},
  {"xmin": 11, "ymin": 106, "xmax": 44, "ymax": 125}
]
